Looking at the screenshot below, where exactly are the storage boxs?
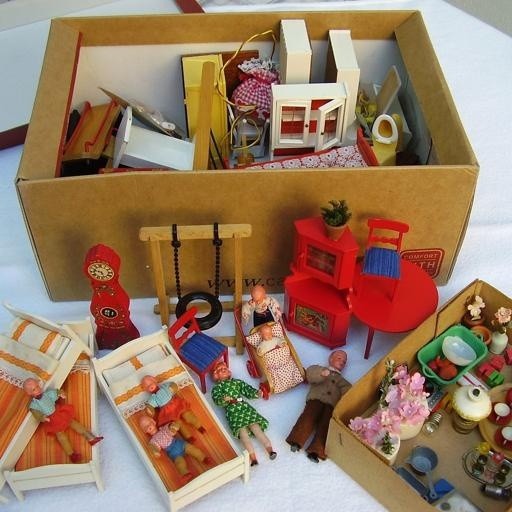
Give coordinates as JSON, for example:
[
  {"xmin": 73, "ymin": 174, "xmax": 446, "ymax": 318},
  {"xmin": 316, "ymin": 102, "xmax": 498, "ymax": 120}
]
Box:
[
  {"xmin": 326, "ymin": 275, "xmax": 512, "ymax": 512},
  {"xmin": 12, "ymin": 9, "xmax": 481, "ymax": 304}
]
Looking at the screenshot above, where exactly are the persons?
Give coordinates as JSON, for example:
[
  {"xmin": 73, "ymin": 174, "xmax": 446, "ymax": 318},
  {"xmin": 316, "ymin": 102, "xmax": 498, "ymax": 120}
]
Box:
[
  {"xmin": 257, "ymin": 326, "xmax": 286, "ymax": 356},
  {"xmin": 23, "ymin": 378, "xmax": 105, "ymax": 464},
  {"xmin": 241, "ymin": 285, "xmax": 281, "ymax": 328},
  {"xmin": 210, "ymin": 361, "xmax": 277, "ymax": 466},
  {"xmin": 139, "ymin": 375, "xmax": 216, "ymax": 483},
  {"xmin": 285, "ymin": 350, "xmax": 352, "ymax": 463}
]
